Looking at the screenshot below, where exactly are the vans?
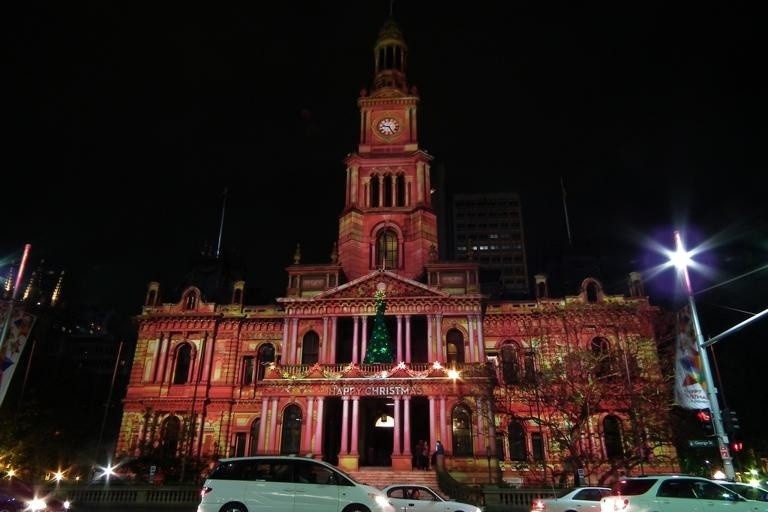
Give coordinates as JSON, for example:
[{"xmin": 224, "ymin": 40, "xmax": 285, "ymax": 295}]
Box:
[{"xmin": 195, "ymin": 455, "xmax": 396, "ymax": 512}]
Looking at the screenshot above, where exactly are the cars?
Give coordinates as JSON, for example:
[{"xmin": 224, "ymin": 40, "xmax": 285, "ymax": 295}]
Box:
[
  {"xmin": 532, "ymin": 486, "xmax": 615, "ymax": 512},
  {"xmin": 380, "ymin": 484, "xmax": 483, "ymax": 512}
]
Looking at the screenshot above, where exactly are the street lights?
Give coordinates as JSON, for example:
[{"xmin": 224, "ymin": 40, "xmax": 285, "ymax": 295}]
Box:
[{"xmin": 667, "ymin": 230, "xmax": 737, "ymax": 483}]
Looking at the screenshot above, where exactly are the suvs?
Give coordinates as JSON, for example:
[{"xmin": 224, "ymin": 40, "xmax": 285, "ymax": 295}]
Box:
[{"xmin": 599, "ymin": 473, "xmax": 768, "ymax": 512}]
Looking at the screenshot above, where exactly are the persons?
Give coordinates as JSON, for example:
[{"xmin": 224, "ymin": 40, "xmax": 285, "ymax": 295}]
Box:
[
  {"xmin": 411, "ymin": 490, "xmax": 418, "ymax": 500},
  {"xmin": 620, "ymin": 471, "xmax": 626, "ymax": 477},
  {"xmin": 418, "ymin": 490, "xmax": 420, "ymax": 500},
  {"xmin": 416, "ymin": 438, "xmax": 443, "ymax": 471}
]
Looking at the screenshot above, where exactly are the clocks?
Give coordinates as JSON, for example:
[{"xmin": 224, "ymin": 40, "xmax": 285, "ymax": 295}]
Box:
[{"xmin": 372, "ymin": 114, "xmax": 405, "ymax": 143}]
